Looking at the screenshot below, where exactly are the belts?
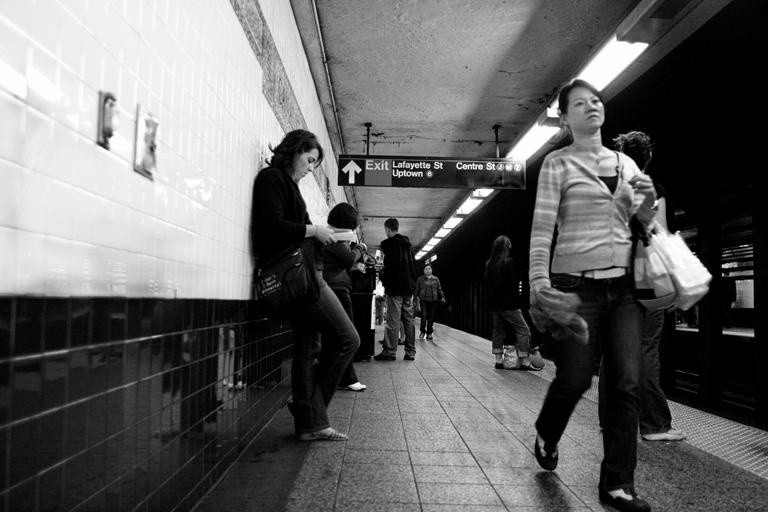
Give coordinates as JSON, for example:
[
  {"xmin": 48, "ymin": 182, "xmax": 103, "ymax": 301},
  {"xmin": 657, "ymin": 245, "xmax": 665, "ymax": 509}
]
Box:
[{"xmin": 568, "ymin": 267, "xmax": 633, "ymax": 281}]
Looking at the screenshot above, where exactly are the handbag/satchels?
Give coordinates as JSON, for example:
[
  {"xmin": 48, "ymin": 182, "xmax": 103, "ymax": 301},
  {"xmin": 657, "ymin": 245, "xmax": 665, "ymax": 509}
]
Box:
[
  {"xmin": 252, "ymin": 243, "xmax": 321, "ymax": 322},
  {"xmin": 627, "ymin": 214, "xmax": 678, "ymax": 314},
  {"xmin": 503, "ymin": 343, "xmax": 546, "ymax": 372},
  {"xmin": 647, "ymin": 225, "xmax": 715, "ymax": 312}
]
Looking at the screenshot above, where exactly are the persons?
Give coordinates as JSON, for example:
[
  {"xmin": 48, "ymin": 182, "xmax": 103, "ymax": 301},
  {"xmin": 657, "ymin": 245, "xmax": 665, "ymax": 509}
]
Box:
[
  {"xmin": 480, "ymin": 234, "xmax": 545, "ymax": 375},
  {"xmin": 614, "ymin": 126, "xmax": 693, "ymax": 443},
  {"xmin": 528, "ymin": 77, "xmax": 665, "ymax": 510},
  {"xmin": 319, "ymin": 199, "xmax": 369, "ymax": 396},
  {"xmin": 412, "ymin": 263, "xmax": 444, "ymax": 339},
  {"xmin": 375, "ymin": 217, "xmax": 416, "ymax": 365},
  {"xmin": 249, "ymin": 128, "xmax": 360, "ymax": 442},
  {"xmin": 378, "ymin": 304, "xmax": 407, "ymax": 346}
]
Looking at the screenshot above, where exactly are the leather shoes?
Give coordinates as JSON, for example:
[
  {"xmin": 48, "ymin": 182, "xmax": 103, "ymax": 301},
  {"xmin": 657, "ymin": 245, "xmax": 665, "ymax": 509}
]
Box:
[
  {"xmin": 599, "ymin": 487, "xmax": 651, "ymax": 512},
  {"xmin": 535, "ymin": 436, "xmax": 561, "ymax": 470},
  {"xmin": 519, "ymin": 362, "xmax": 543, "ymax": 371},
  {"xmin": 494, "ymin": 362, "xmax": 504, "ymax": 369},
  {"xmin": 374, "ymin": 353, "xmax": 396, "ymax": 361},
  {"xmin": 403, "ymin": 354, "xmax": 416, "ymax": 360}
]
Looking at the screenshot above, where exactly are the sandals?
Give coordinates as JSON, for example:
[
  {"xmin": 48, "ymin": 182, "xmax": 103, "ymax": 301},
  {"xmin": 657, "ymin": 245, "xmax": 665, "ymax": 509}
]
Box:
[{"xmin": 297, "ymin": 426, "xmax": 349, "ymax": 442}]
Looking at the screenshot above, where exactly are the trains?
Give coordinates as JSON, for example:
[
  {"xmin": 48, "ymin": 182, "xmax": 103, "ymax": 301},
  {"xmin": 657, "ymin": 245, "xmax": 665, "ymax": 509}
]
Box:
[{"xmin": 445, "ymin": 176, "xmax": 767, "ymax": 435}]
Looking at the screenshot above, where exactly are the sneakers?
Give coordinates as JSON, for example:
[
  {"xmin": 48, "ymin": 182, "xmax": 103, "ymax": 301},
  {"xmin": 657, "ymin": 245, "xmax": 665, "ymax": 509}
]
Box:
[
  {"xmin": 640, "ymin": 427, "xmax": 688, "ymax": 441},
  {"xmin": 348, "ymin": 380, "xmax": 367, "ymax": 392},
  {"xmin": 419, "ymin": 332, "xmax": 425, "ymax": 338},
  {"xmin": 426, "ymin": 333, "xmax": 433, "ymax": 340}
]
